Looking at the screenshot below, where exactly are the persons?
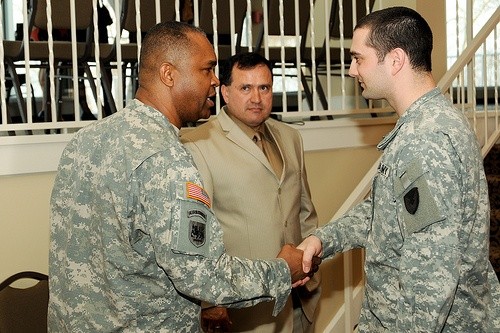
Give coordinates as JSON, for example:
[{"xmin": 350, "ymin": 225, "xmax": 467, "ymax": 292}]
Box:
[
  {"xmin": 45, "ymin": 20, "xmax": 321, "ymax": 333},
  {"xmin": 176, "ymin": 51, "xmax": 323, "ymax": 333},
  {"xmin": 292, "ymin": 7, "xmax": 500, "ymax": 333}
]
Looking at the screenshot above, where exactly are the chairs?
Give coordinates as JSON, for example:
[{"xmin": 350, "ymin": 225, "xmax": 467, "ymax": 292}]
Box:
[
  {"xmin": 0, "ymin": 271, "xmax": 49, "ymax": 333},
  {"xmin": 0, "ymin": 0, "xmax": 104, "ymax": 137},
  {"xmin": 302, "ymin": 1, "xmax": 379, "ymax": 121},
  {"xmin": 186, "ymin": 0, "xmax": 250, "ymax": 126},
  {"xmin": 86, "ymin": 0, "xmax": 177, "ymax": 119}
]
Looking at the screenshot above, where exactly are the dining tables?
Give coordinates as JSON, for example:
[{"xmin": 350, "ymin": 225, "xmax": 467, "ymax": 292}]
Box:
[{"xmin": 237, "ymin": 0, "xmax": 322, "ymax": 122}]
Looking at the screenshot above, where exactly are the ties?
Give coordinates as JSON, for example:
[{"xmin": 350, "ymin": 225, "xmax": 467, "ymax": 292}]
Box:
[{"xmin": 254, "ymin": 132, "xmax": 282, "ymax": 179}]
